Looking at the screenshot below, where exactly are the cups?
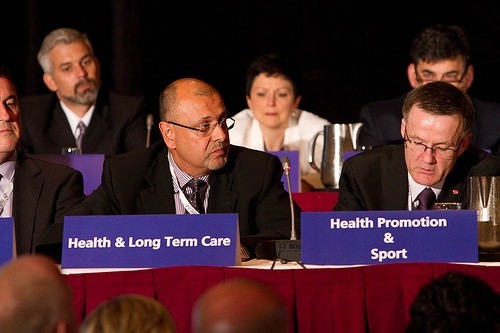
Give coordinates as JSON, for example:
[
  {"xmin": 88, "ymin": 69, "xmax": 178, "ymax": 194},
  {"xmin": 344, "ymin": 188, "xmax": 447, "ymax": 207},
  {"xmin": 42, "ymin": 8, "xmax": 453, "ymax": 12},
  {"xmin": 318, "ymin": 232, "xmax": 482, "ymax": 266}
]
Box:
[
  {"xmin": 435, "ymin": 203, "xmax": 461, "ymax": 211},
  {"xmin": 61, "ymin": 147, "xmax": 79, "ymax": 153}
]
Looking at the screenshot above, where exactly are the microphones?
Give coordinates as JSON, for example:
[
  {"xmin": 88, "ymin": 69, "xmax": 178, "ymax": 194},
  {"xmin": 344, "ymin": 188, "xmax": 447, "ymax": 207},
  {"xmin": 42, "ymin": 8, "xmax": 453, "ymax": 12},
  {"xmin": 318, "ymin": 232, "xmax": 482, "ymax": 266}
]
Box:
[
  {"xmin": 255, "ymin": 155, "xmax": 302, "ymax": 261},
  {"xmin": 145, "ymin": 113, "xmax": 154, "ymax": 149}
]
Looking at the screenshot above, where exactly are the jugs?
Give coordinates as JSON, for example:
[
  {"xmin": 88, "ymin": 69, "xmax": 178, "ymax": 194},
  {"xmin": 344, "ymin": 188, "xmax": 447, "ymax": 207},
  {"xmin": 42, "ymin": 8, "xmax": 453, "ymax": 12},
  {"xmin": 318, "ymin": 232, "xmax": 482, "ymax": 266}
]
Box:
[
  {"xmin": 309, "ymin": 123, "xmax": 361, "ymax": 190},
  {"xmin": 466, "ymin": 176, "xmax": 499, "ymax": 251}
]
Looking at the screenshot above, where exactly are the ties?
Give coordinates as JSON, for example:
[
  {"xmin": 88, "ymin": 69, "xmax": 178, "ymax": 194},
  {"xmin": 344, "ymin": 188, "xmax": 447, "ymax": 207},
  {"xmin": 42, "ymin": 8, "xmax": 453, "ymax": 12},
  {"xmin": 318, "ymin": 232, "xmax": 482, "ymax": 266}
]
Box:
[
  {"xmin": 183, "ymin": 178, "xmax": 208, "ymax": 215},
  {"xmin": 414, "ymin": 188, "xmax": 436, "ymax": 210},
  {"xmin": 77, "ymin": 121, "xmax": 87, "ymax": 148}
]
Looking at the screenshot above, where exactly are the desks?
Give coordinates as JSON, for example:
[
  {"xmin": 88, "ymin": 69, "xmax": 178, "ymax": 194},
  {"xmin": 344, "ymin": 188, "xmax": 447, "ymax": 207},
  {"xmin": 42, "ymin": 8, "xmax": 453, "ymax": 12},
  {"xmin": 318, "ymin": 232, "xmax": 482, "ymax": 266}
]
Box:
[{"xmin": 63, "ymin": 260, "xmax": 500, "ymax": 333}]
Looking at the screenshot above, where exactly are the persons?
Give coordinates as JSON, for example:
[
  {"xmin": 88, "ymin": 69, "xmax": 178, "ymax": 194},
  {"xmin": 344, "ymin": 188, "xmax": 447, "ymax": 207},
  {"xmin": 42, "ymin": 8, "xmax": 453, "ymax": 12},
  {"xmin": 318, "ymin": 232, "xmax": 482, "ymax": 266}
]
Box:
[
  {"xmin": 17, "ymin": 28, "xmax": 145, "ymax": 158},
  {"xmin": 411, "ymin": 269, "xmax": 500, "ymax": 333},
  {"xmin": 35, "ymin": 77, "xmax": 293, "ymax": 264},
  {"xmin": 0, "ymin": 251, "xmax": 75, "ymax": 333},
  {"xmin": 335, "ymin": 81, "xmax": 500, "ymax": 265},
  {"xmin": 189, "ymin": 277, "xmax": 287, "ymax": 333},
  {"xmin": 361, "ymin": 20, "xmax": 500, "ymax": 147},
  {"xmin": 79, "ymin": 294, "xmax": 174, "ymax": 333},
  {"xmin": 0, "ymin": 64, "xmax": 84, "ymax": 267},
  {"xmin": 225, "ymin": 54, "xmax": 331, "ymax": 175}
]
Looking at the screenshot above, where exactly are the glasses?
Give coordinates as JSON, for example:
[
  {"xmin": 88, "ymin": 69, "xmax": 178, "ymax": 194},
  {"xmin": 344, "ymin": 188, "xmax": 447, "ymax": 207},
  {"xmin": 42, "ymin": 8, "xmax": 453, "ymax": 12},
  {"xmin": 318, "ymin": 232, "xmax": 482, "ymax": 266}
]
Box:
[
  {"xmin": 415, "ymin": 64, "xmax": 468, "ymax": 87},
  {"xmin": 167, "ymin": 117, "xmax": 235, "ymax": 138},
  {"xmin": 404, "ymin": 126, "xmax": 464, "ymax": 160}
]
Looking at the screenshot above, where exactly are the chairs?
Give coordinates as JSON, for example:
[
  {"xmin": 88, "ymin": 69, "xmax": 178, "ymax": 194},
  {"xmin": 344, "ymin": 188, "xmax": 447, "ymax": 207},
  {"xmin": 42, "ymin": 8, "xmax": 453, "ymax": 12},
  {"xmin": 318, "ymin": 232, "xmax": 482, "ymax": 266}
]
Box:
[{"xmin": 20, "ymin": 153, "xmax": 106, "ymax": 195}]
